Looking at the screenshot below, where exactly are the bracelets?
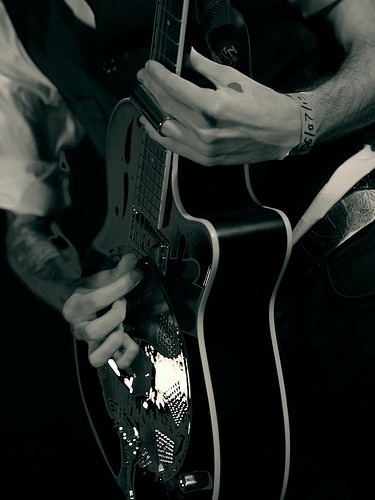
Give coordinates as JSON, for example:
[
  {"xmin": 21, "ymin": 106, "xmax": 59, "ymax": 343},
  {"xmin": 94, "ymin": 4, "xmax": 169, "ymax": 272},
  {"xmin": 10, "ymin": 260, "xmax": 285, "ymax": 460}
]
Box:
[{"xmin": 285, "ymin": 91, "xmax": 317, "ymax": 157}]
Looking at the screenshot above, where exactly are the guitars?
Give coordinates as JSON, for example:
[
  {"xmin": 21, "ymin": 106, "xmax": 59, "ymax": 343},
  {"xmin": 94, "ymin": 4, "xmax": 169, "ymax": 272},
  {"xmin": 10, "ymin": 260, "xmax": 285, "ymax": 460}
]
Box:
[{"xmin": 64, "ymin": 0, "xmax": 290, "ymax": 500}]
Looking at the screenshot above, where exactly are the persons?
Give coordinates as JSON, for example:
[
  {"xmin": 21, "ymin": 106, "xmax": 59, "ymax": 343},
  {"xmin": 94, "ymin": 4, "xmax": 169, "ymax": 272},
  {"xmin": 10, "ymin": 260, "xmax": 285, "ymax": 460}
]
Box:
[{"xmin": 1, "ymin": 1, "xmax": 375, "ymax": 499}]
